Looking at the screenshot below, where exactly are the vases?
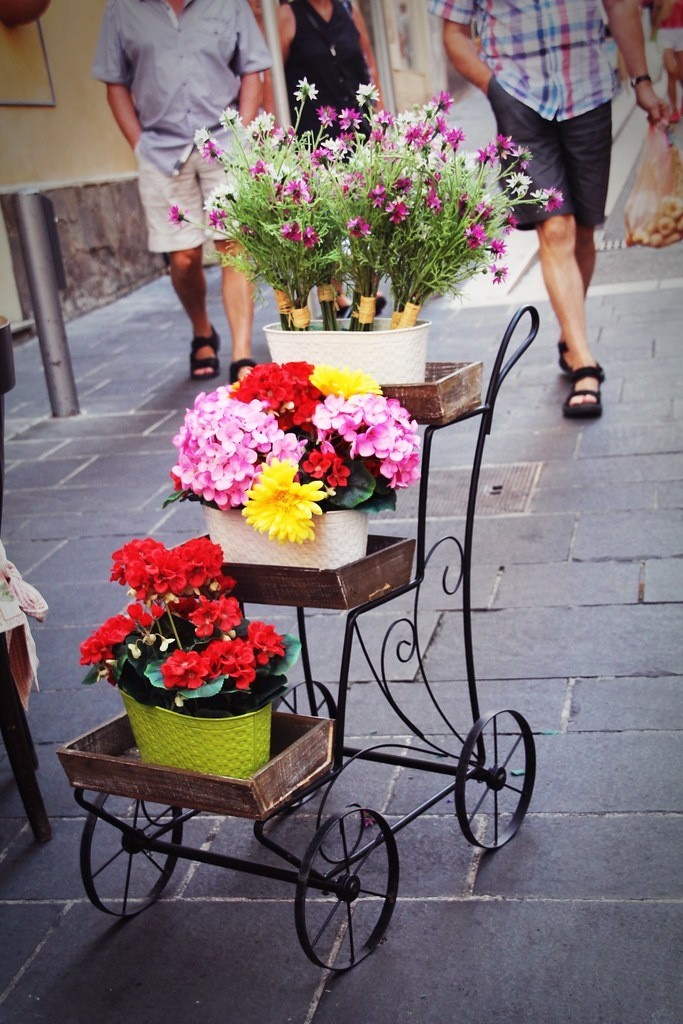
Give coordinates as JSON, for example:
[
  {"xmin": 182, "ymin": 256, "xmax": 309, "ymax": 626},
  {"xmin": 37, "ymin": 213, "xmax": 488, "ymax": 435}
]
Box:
[
  {"xmin": 200, "ymin": 504, "xmax": 371, "ymax": 572},
  {"xmin": 116, "ymin": 686, "xmax": 274, "ymax": 779},
  {"xmin": 262, "ymin": 317, "xmax": 432, "ymax": 385}
]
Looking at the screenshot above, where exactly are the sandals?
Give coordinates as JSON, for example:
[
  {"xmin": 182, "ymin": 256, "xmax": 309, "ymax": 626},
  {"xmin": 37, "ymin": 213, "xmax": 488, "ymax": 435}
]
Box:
[
  {"xmin": 189, "ymin": 326, "xmax": 219, "ymax": 379},
  {"xmin": 228, "ymin": 358, "xmax": 257, "ymax": 385},
  {"xmin": 558, "ymin": 342, "xmax": 606, "ymax": 417}
]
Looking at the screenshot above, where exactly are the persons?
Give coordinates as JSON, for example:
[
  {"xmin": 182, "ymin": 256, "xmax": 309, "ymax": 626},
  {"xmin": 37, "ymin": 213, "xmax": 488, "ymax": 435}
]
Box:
[
  {"xmin": 260, "ymin": 1, "xmax": 386, "ymax": 318},
  {"xmin": 427, "ymin": 0, "xmax": 671, "ymax": 416},
  {"xmin": 91, "ymin": 1, "xmax": 272, "ymax": 388},
  {"xmin": 657, "ymin": 1, "xmax": 683, "ymax": 123}
]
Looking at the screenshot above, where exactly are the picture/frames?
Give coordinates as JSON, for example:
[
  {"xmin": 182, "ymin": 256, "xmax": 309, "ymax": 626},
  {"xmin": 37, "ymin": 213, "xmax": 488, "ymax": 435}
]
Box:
[{"xmin": 0, "ymin": 18, "xmax": 56, "ymax": 107}]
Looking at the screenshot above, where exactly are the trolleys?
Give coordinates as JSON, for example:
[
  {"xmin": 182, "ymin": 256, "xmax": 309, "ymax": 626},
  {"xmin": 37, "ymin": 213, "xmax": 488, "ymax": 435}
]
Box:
[{"xmin": 59, "ymin": 303, "xmax": 540, "ymax": 973}]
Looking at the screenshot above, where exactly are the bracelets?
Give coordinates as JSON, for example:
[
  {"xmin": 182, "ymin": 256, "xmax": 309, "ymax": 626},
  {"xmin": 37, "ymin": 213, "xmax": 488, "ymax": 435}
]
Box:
[{"xmin": 631, "ymin": 75, "xmax": 651, "ymax": 87}]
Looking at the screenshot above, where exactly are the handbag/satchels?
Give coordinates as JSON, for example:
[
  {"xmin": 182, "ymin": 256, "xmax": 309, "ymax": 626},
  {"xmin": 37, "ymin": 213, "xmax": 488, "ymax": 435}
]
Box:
[{"xmin": 624, "ymin": 119, "xmax": 683, "ymax": 248}]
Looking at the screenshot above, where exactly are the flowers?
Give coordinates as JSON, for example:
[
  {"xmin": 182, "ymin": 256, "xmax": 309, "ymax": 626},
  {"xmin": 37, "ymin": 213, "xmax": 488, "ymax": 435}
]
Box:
[
  {"xmin": 169, "ymin": 75, "xmax": 564, "ymax": 332},
  {"xmin": 78, "ymin": 536, "xmax": 302, "ymax": 722},
  {"xmin": 162, "ymin": 360, "xmax": 423, "ymax": 547}
]
